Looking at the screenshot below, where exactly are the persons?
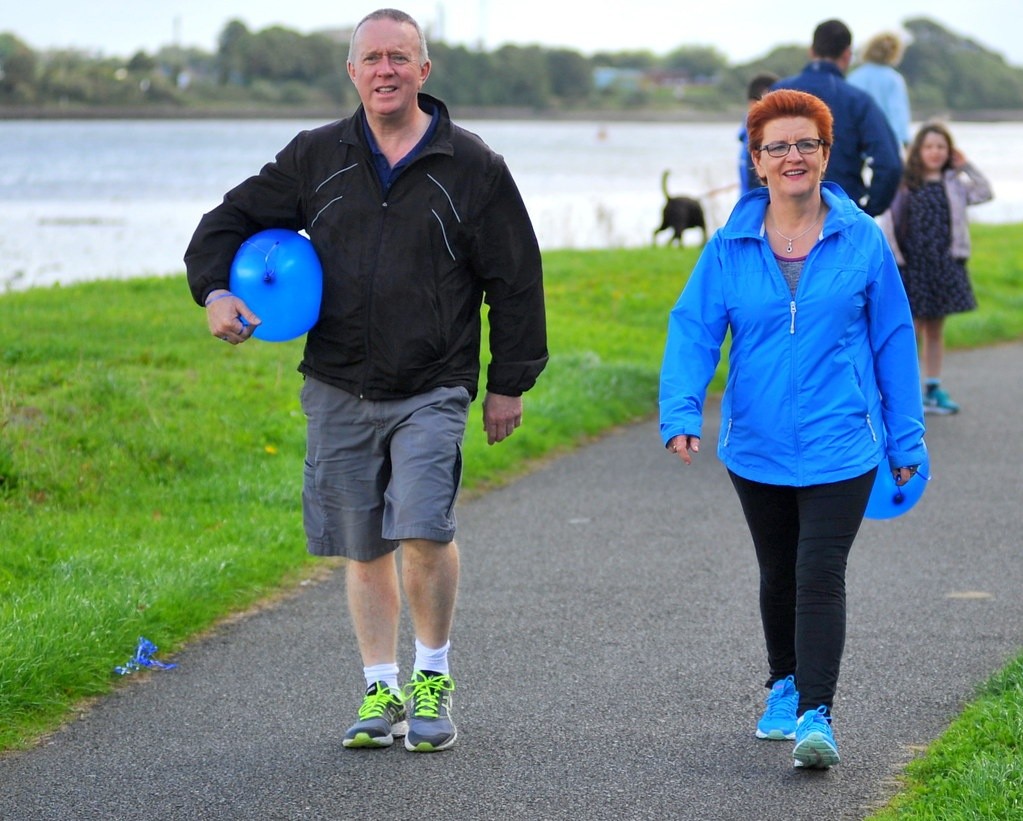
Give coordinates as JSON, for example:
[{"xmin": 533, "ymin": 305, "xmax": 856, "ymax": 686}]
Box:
[
  {"xmin": 841, "ymin": 31, "xmax": 911, "ymax": 151},
  {"xmin": 769, "ymin": 16, "xmax": 908, "ymax": 222},
  {"xmin": 181, "ymin": 10, "xmax": 554, "ymax": 758},
  {"xmin": 738, "ymin": 72, "xmax": 779, "ymax": 196},
  {"xmin": 880, "ymin": 119, "xmax": 992, "ymax": 420},
  {"xmin": 657, "ymin": 87, "xmax": 930, "ymax": 770}
]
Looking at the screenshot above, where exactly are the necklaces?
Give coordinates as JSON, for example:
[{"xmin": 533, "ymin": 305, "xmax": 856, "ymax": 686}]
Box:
[{"xmin": 768, "ymin": 209, "xmax": 823, "ymax": 255}]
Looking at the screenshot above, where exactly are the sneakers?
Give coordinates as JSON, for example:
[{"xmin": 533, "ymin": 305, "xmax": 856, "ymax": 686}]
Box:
[
  {"xmin": 922, "ymin": 387, "xmax": 960, "ymax": 418},
  {"xmin": 342, "ymin": 680, "xmax": 408, "ymax": 748},
  {"xmin": 793, "ymin": 710, "xmax": 841, "ymax": 770},
  {"xmin": 404, "ymin": 669, "xmax": 458, "ymax": 752},
  {"xmin": 755, "ymin": 679, "xmax": 799, "ymax": 741}
]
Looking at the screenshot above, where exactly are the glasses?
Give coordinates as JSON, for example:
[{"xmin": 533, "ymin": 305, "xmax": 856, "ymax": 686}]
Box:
[{"xmin": 758, "ymin": 137, "xmax": 831, "ymax": 157}]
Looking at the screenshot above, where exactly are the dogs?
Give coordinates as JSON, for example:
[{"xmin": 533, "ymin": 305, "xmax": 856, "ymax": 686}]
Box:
[{"xmin": 651, "ymin": 171, "xmax": 707, "ymax": 247}]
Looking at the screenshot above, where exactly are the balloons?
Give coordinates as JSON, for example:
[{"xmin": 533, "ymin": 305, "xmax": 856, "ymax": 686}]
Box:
[
  {"xmin": 861, "ymin": 437, "xmax": 929, "ymax": 520},
  {"xmin": 228, "ymin": 228, "xmax": 324, "ymax": 342}
]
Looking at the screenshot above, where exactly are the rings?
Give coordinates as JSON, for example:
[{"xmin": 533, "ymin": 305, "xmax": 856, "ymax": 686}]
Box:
[{"xmin": 672, "ymin": 446, "xmax": 676, "ymax": 450}]
